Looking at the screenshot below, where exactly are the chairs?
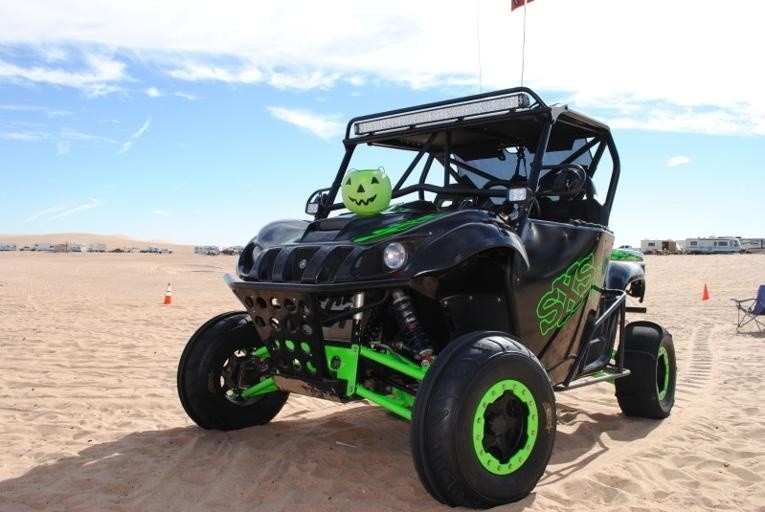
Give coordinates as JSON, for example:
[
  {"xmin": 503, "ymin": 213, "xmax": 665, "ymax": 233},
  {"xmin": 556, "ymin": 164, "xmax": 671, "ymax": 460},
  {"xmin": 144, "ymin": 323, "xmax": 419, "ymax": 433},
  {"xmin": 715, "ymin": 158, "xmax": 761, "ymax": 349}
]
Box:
[{"xmin": 729, "ymin": 284, "xmax": 765, "ymax": 336}]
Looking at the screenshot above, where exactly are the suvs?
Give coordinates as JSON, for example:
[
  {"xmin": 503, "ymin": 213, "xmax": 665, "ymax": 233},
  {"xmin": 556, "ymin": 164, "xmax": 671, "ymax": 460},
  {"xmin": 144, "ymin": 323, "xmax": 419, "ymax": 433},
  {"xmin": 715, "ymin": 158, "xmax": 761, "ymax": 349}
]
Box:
[{"xmin": 175, "ymin": 85, "xmax": 678, "ymax": 509}]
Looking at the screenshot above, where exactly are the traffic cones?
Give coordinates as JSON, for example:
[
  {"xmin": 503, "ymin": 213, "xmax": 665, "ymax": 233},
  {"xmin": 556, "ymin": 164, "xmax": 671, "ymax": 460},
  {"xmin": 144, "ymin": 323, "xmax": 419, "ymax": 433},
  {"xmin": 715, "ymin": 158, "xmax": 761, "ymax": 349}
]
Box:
[
  {"xmin": 164, "ymin": 281, "xmax": 173, "ymax": 304},
  {"xmin": 703, "ymin": 283, "xmax": 710, "ymax": 301}
]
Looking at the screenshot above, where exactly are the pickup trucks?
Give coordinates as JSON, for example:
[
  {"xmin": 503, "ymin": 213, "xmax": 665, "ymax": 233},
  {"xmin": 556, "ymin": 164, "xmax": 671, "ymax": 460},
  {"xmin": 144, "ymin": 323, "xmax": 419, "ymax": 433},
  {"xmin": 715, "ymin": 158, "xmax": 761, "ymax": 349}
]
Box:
[{"xmin": 158, "ymin": 247, "xmax": 174, "ymax": 254}]
